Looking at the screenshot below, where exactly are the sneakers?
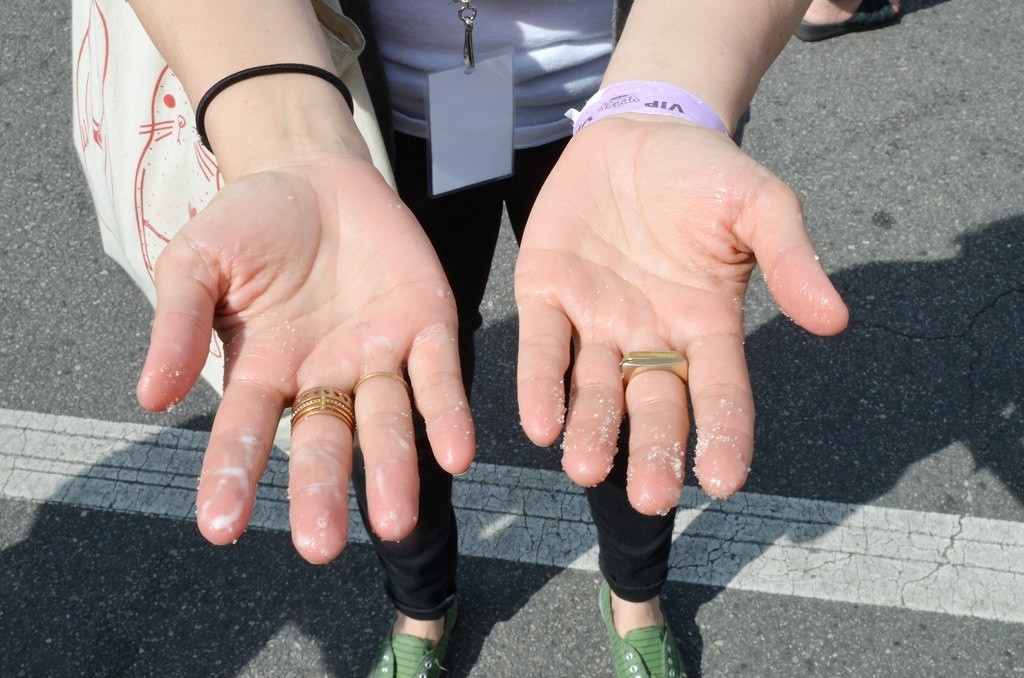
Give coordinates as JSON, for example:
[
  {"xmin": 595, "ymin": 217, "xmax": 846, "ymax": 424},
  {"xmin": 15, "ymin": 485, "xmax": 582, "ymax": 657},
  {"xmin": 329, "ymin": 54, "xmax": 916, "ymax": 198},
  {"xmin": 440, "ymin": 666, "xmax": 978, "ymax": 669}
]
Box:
[
  {"xmin": 598, "ymin": 579, "xmax": 684, "ymax": 678},
  {"xmin": 366, "ymin": 597, "xmax": 458, "ymax": 678}
]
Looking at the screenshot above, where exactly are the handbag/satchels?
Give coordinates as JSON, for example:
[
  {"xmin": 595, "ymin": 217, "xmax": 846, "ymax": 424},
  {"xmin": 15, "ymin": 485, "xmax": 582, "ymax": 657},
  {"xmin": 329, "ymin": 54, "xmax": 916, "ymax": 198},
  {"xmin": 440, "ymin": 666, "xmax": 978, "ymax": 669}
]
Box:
[{"xmin": 71, "ymin": 0, "xmax": 400, "ymax": 456}]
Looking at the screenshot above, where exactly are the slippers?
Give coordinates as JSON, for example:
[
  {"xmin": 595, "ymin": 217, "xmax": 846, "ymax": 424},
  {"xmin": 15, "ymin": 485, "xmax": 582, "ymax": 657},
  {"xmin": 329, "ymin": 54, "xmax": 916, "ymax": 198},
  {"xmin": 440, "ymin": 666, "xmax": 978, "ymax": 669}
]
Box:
[{"xmin": 795, "ymin": 0, "xmax": 900, "ymax": 42}]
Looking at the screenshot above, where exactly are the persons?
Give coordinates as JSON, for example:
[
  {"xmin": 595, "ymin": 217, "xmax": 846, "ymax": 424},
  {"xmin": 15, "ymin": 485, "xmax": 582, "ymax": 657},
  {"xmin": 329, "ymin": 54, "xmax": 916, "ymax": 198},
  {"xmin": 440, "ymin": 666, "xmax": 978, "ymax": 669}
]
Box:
[
  {"xmin": 126, "ymin": 0, "xmax": 849, "ymax": 677},
  {"xmin": 795, "ymin": 0, "xmax": 902, "ymax": 42}
]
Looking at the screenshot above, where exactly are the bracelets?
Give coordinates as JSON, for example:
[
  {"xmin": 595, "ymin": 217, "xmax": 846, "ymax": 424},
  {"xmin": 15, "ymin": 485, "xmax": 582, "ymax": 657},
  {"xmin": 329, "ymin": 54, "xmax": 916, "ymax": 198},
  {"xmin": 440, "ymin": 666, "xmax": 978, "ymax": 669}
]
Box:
[
  {"xmin": 565, "ymin": 81, "xmax": 727, "ymax": 133},
  {"xmin": 196, "ymin": 63, "xmax": 354, "ymax": 155}
]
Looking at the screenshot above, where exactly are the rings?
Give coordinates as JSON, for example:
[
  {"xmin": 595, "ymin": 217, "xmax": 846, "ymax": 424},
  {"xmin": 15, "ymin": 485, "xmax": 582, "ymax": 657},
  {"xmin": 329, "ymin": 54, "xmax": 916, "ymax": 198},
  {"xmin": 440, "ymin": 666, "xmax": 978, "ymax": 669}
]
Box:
[
  {"xmin": 291, "ymin": 386, "xmax": 355, "ymax": 437},
  {"xmin": 621, "ymin": 351, "xmax": 687, "ymax": 383},
  {"xmin": 352, "ymin": 371, "xmax": 411, "ymax": 397}
]
